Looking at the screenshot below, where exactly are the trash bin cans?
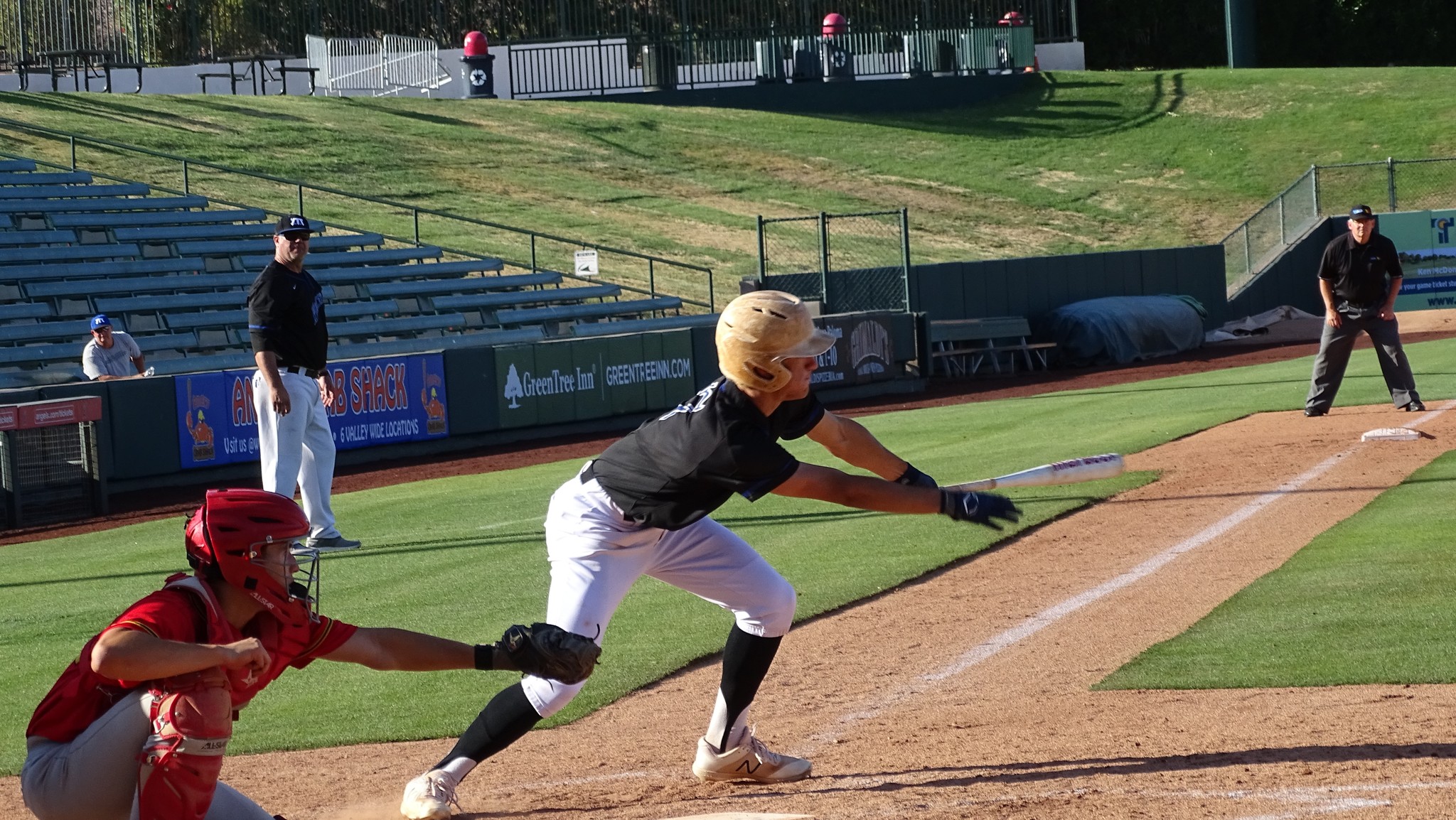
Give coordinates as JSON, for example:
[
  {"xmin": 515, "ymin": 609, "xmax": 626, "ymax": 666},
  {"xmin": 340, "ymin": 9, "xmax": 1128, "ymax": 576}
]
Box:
[{"xmin": 641, "ymin": 42, "xmax": 679, "ymax": 84}]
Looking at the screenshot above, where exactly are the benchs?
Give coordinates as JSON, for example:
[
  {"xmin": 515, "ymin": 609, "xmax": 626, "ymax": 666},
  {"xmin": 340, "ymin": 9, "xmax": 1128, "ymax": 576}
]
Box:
[
  {"xmin": 931, "ymin": 316, "xmax": 1057, "ymax": 379},
  {"xmin": 10, "ymin": 59, "xmax": 35, "ymax": 92},
  {"xmin": 1, "ymin": 157, "xmax": 722, "ymax": 391},
  {"xmin": 94, "ymin": 61, "xmax": 145, "ymax": 93},
  {"xmin": 271, "ymin": 66, "xmax": 319, "ymax": 96},
  {"xmin": 194, "ymin": 73, "xmax": 245, "ymax": 95}
]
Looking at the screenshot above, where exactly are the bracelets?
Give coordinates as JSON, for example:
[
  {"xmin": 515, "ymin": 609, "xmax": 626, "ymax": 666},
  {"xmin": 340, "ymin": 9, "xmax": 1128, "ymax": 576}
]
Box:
[{"xmin": 317, "ymin": 370, "xmax": 329, "ymax": 378}]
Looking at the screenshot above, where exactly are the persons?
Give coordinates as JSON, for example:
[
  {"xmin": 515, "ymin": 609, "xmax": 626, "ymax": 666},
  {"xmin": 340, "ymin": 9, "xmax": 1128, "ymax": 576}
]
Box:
[
  {"xmin": 396, "ymin": 293, "xmax": 1020, "ymax": 818},
  {"xmin": 1303, "ymin": 204, "xmax": 1425, "ymax": 417},
  {"xmin": 17, "ymin": 489, "xmax": 599, "ymax": 820},
  {"xmin": 246, "ymin": 214, "xmax": 362, "ymax": 555},
  {"xmin": 83, "ymin": 314, "xmax": 146, "ymax": 380}
]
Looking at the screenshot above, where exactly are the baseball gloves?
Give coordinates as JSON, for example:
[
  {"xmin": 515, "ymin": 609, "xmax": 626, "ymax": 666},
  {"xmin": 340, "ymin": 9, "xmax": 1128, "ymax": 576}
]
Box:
[{"xmin": 474, "ymin": 623, "xmax": 602, "ymax": 692}]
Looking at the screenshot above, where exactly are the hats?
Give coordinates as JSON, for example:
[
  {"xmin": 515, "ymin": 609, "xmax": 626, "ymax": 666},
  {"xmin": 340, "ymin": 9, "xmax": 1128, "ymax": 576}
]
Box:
[
  {"xmin": 1350, "ymin": 204, "xmax": 1374, "ymax": 223},
  {"xmin": 90, "ymin": 314, "xmax": 112, "ymax": 332},
  {"xmin": 272, "ymin": 213, "xmax": 315, "ymax": 241}
]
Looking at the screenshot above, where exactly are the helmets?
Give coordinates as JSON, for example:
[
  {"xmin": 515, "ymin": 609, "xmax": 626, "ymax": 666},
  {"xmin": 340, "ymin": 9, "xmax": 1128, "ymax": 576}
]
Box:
[
  {"xmin": 711, "ymin": 286, "xmax": 837, "ymax": 391},
  {"xmin": 183, "ymin": 487, "xmax": 313, "ymax": 574}
]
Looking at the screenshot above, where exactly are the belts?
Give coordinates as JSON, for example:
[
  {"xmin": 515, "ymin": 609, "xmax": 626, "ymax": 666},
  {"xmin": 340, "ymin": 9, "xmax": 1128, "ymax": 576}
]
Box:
[{"xmin": 274, "ymin": 364, "xmax": 324, "ymax": 379}]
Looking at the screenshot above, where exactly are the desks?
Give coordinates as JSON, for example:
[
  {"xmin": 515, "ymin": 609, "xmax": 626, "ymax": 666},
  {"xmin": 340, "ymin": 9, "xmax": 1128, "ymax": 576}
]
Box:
[
  {"xmin": 217, "ymin": 54, "xmax": 297, "ymax": 95},
  {"xmin": 36, "ymin": 49, "xmax": 121, "ymax": 92}
]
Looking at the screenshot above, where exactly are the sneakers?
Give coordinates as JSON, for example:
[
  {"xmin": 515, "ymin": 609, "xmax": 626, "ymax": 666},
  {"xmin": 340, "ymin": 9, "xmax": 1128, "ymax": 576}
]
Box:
[
  {"xmin": 1303, "ymin": 403, "xmax": 1323, "ymax": 418},
  {"xmin": 396, "ymin": 763, "xmax": 456, "ymax": 820},
  {"xmin": 303, "ymin": 531, "xmax": 364, "ymax": 555},
  {"xmin": 1403, "ymin": 399, "xmax": 1426, "ymax": 412},
  {"xmin": 694, "ymin": 727, "xmax": 815, "ymax": 786},
  {"xmin": 285, "ymin": 538, "xmax": 322, "ymax": 559}
]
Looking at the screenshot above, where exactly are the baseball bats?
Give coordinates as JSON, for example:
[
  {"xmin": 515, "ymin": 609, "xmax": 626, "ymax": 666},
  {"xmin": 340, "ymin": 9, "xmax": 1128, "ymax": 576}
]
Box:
[{"xmin": 942, "ymin": 450, "xmax": 1128, "ymax": 492}]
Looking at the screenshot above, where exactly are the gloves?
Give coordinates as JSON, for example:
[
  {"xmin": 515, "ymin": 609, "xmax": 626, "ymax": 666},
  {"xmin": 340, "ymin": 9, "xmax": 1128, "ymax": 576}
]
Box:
[
  {"xmin": 935, "ymin": 486, "xmax": 1026, "ymax": 536},
  {"xmin": 894, "ymin": 461, "xmax": 956, "ymax": 494}
]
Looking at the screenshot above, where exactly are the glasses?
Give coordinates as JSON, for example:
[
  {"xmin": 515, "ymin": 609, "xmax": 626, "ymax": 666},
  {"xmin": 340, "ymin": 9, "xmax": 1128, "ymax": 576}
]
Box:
[
  {"xmin": 276, "ymin": 230, "xmax": 315, "ymax": 244},
  {"xmin": 92, "ymin": 326, "xmax": 112, "ymax": 334}
]
